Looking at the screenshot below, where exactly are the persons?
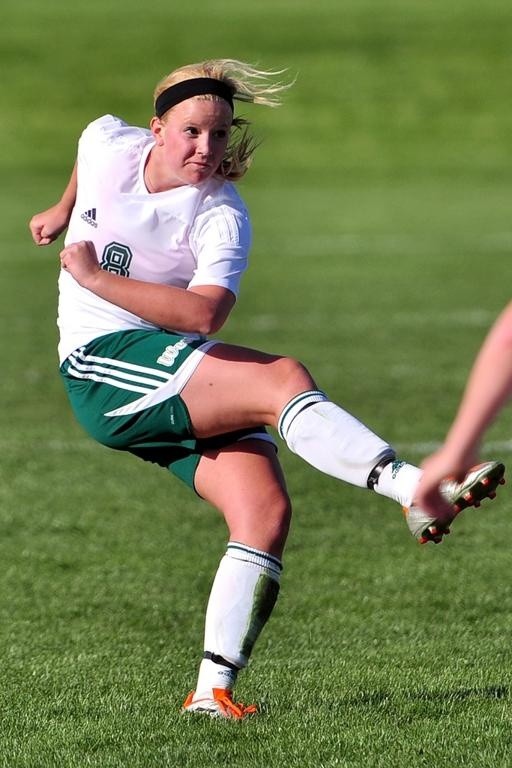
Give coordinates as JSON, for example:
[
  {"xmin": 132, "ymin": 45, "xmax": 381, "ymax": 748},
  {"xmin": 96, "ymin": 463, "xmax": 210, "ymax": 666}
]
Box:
[
  {"xmin": 26, "ymin": 57, "xmax": 509, "ymax": 720},
  {"xmin": 408, "ymin": 287, "xmax": 510, "ymax": 529}
]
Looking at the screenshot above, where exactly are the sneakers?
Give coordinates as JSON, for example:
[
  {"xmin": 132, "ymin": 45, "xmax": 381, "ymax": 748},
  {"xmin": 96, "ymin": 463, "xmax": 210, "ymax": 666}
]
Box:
[
  {"xmin": 402, "ymin": 459, "xmax": 505, "ymax": 545},
  {"xmin": 177, "ymin": 689, "xmax": 259, "ymax": 721}
]
